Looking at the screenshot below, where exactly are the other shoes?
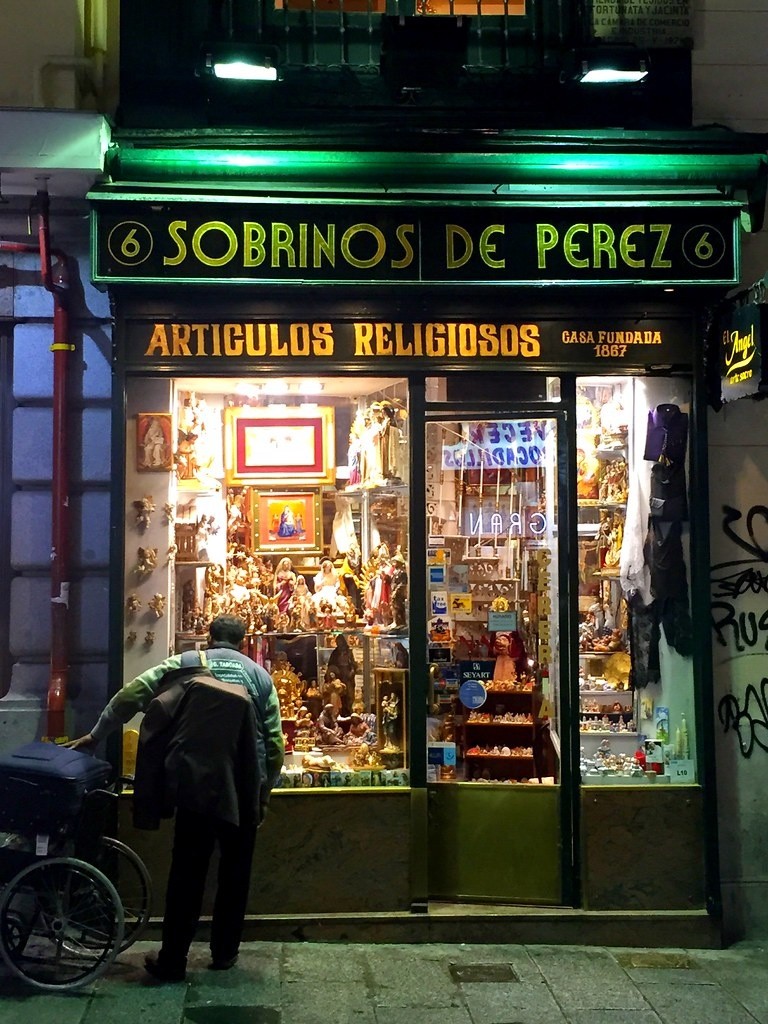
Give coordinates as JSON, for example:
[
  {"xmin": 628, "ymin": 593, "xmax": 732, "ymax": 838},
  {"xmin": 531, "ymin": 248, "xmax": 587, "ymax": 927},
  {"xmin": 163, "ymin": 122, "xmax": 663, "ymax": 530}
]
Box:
[
  {"xmin": 213, "ymin": 955, "xmax": 239, "ymax": 970},
  {"xmin": 145, "ymin": 950, "xmax": 185, "ymax": 980}
]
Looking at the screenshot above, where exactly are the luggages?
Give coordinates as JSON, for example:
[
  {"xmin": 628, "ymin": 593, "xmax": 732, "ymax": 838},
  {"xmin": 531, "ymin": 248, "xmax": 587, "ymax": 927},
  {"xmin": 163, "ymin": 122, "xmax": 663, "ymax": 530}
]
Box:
[{"xmin": 0, "ymin": 739, "xmax": 113, "ymax": 836}]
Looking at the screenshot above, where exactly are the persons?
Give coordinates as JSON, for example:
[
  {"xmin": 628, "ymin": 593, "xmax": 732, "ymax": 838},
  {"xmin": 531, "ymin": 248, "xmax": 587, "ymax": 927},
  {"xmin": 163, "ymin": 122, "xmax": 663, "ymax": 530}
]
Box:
[
  {"xmin": 579, "ymin": 458, "xmax": 630, "ymax": 654},
  {"xmin": 127, "ymin": 403, "xmax": 407, "ymax": 751},
  {"xmin": 56, "ymin": 615, "xmax": 284, "ymax": 988}
]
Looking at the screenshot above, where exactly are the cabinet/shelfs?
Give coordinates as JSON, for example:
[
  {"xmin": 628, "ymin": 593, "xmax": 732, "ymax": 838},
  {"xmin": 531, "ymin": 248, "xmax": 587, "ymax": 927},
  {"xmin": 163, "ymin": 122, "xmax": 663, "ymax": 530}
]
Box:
[{"xmin": 176, "ymin": 389, "xmax": 670, "ymax": 785}]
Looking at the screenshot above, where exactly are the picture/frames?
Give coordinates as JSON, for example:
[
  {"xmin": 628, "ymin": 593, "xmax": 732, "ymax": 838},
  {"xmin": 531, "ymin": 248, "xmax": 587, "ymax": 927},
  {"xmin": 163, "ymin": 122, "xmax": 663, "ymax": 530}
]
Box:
[
  {"xmin": 248, "ymin": 485, "xmax": 325, "ymax": 556},
  {"xmin": 222, "ymin": 404, "xmax": 337, "ymax": 487}
]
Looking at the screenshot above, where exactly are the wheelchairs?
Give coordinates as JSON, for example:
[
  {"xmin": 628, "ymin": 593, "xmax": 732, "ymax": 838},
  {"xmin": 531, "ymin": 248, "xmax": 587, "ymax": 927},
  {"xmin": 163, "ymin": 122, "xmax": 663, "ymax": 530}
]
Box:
[{"xmin": 0, "ymin": 775, "xmax": 156, "ymax": 991}]
